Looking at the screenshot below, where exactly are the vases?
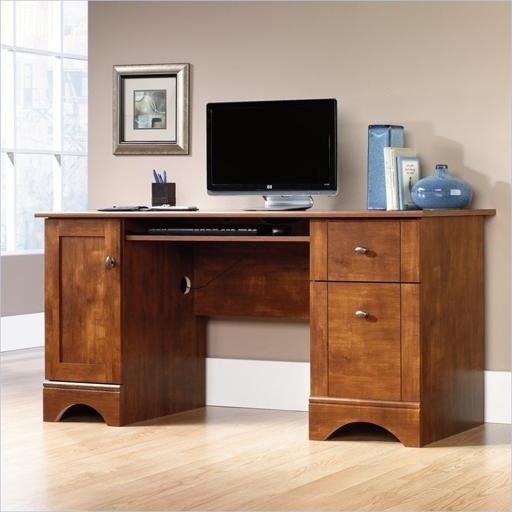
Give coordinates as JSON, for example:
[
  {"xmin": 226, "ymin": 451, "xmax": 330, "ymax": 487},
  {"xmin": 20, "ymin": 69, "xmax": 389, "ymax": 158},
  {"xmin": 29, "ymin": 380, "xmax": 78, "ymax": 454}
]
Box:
[
  {"xmin": 367, "ymin": 124, "xmax": 405, "ymax": 210},
  {"xmin": 410, "ymin": 164, "xmax": 472, "ymax": 209}
]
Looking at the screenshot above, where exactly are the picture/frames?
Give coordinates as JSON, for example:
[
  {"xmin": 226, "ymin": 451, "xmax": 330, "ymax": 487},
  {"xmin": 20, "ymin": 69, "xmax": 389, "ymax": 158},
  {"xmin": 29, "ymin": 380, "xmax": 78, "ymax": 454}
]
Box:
[{"xmin": 111, "ymin": 63, "xmax": 190, "ymax": 156}]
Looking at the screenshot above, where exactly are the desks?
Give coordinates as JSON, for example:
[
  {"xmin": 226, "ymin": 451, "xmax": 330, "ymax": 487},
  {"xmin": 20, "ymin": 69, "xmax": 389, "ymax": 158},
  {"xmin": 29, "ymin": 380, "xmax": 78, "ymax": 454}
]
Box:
[{"xmin": 34, "ymin": 207, "xmax": 500, "ymax": 447}]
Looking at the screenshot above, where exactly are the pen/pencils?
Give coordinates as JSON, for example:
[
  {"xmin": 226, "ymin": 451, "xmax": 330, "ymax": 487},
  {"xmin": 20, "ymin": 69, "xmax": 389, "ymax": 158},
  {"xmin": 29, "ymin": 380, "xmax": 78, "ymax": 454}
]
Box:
[{"xmin": 153, "ymin": 169, "xmax": 167, "ymax": 184}]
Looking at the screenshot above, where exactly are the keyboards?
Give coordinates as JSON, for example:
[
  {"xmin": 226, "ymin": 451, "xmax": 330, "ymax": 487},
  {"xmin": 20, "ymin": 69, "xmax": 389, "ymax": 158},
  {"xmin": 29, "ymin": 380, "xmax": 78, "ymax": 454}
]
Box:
[{"xmin": 133, "ymin": 224, "xmax": 262, "ymax": 235}]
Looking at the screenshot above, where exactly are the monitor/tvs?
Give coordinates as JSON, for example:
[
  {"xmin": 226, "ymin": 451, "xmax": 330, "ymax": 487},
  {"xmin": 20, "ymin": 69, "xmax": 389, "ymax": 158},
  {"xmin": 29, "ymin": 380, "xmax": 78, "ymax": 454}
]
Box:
[{"xmin": 206, "ymin": 97, "xmax": 338, "ymax": 210}]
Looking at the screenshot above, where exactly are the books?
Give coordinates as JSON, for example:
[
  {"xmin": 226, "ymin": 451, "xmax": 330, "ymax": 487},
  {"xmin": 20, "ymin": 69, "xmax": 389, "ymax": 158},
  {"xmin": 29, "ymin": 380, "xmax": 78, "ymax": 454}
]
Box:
[{"xmin": 382, "ymin": 145, "xmax": 423, "ymax": 212}]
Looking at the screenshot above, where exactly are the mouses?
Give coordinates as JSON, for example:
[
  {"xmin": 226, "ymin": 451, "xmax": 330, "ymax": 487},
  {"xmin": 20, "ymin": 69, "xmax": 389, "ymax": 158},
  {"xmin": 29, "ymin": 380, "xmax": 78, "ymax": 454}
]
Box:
[{"xmin": 271, "ymin": 225, "xmax": 289, "ymax": 235}]
